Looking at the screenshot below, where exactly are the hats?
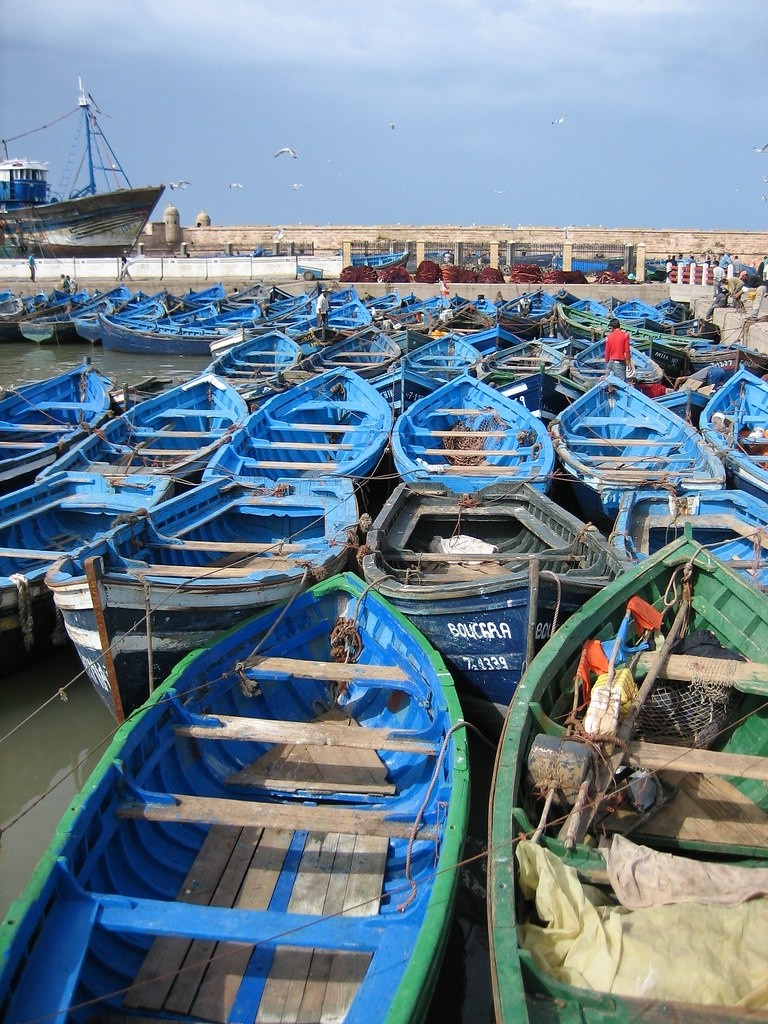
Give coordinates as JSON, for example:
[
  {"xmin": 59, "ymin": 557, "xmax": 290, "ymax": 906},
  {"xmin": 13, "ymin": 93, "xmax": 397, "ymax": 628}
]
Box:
[{"xmin": 739, "ymin": 271, "xmax": 747, "ymax": 278}]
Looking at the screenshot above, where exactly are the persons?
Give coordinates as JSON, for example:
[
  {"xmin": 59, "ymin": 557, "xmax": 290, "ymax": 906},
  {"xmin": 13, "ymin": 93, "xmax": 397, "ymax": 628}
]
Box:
[
  {"xmin": 28, "ymin": 251, "xmax": 37, "ymax": 283},
  {"xmin": 120, "ymin": 248, "xmax": 134, "ymax": 281},
  {"xmin": 665, "ymin": 248, "xmax": 768, "ymax": 320},
  {"xmin": 364, "ymin": 292, "xmax": 370, "ymax": 300},
  {"xmin": 674, "ymin": 365, "xmax": 726, "ymax": 398},
  {"xmin": 605, "ymin": 318, "xmax": 632, "ymax": 382},
  {"xmin": 551, "ymin": 253, "xmax": 558, "ymax": 270},
  {"xmin": 60, "ymin": 274, "xmax": 77, "ymax": 295},
  {"xmin": 316, "ymin": 287, "xmax": 331, "ymax": 328}
]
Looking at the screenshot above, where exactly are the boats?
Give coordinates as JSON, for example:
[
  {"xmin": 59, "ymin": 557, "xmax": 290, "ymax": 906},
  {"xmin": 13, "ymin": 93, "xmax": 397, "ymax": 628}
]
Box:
[
  {"xmin": 361, "ymin": 479, "xmax": 634, "ymax": 707},
  {"xmin": 0, "ymin": 461, "xmax": 175, "ymax": 682},
  {"xmin": 367, "ymin": 330, "xmax": 483, "ymax": 416},
  {"xmin": 283, "ymin": 325, "xmax": 402, "ymax": 383},
  {"xmin": 201, "ymin": 329, "xmax": 304, "ymax": 409},
  {"xmin": 481, "ymin": 336, "xmax": 585, "ymax": 427},
  {"xmin": 606, "ymin": 489, "xmax": 768, "ymax": 594},
  {"xmin": 0, "ymin": 356, "xmax": 173, "ymax": 497},
  {"xmin": 0, "ymin": 281, "xmax": 768, "ymax": 383},
  {"xmin": 35, "ymin": 372, "xmax": 248, "ymax": 492},
  {"xmin": 486, "ymin": 523, "xmax": 768, "ymax": 1024},
  {"xmin": 392, "ymin": 367, "xmax": 555, "ymax": 501},
  {"xmin": 201, "ymin": 366, "xmax": 393, "ymax": 488},
  {"xmin": 209, "ymin": 247, "xmax": 757, "ymax": 281},
  {"xmin": 0, "ymin": 73, "xmax": 166, "ymax": 257},
  {"xmin": 571, "ymin": 336, "xmax": 712, "ymax": 431},
  {"xmin": 0, "ymin": 569, "xmax": 469, "ymax": 1024},
  {"xmin": 43, "ymin": 475, "xmax": 360, "ymax": 726},
  {"xmin": 700, "ymin": 364, "xmax": 768, "ymax": 504},
  {"xmin": 551, "ymin": 372, "xmax": 728, "ymax": 531}
]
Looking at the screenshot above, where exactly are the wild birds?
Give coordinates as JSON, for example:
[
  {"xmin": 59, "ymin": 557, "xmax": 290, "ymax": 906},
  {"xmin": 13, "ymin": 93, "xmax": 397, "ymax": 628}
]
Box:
[
  {"xmin": 169, "ymin": 181, "xmax": 191, "ymax": 191},
  {"xmin": 390, "ymin": 122, "xmax": 398, "ymax": 129},
  {"xmin": 292, "ymin": 183, "xmax": 304, "ymax": 190},
  {"xmin": 229, "ymin": 184, "xmax": 244, "ymax": 189},
  {"xmin": 551, "ymin": 115, "xmax": 568, "ymax": 123},
  {"xmin": 274, "ymin": 148, "xmax": 299, "ymax": 160}
]
